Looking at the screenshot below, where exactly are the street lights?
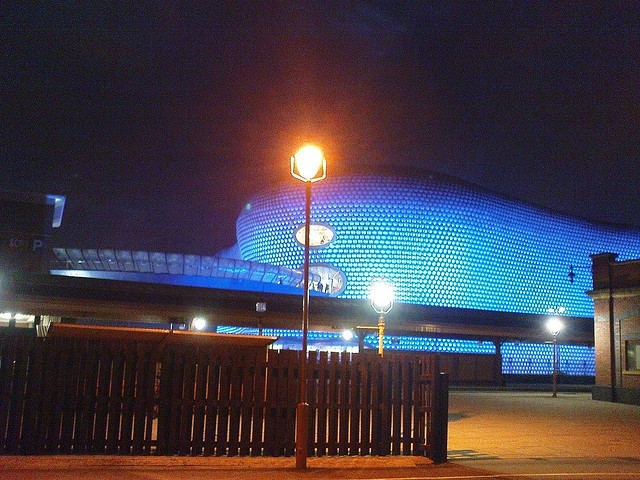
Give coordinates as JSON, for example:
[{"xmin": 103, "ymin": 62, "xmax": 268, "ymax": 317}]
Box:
[
  {"xmin": 546, "ymin": 317, "xmax": 564, "ymax": 397},
  {"xmin": 290, "ymin": 143, "xmax": 327, "ymax": 469},
  {"xmin": 369, "ymin": 277, "xmax": 395, "ymax": 358}
]
